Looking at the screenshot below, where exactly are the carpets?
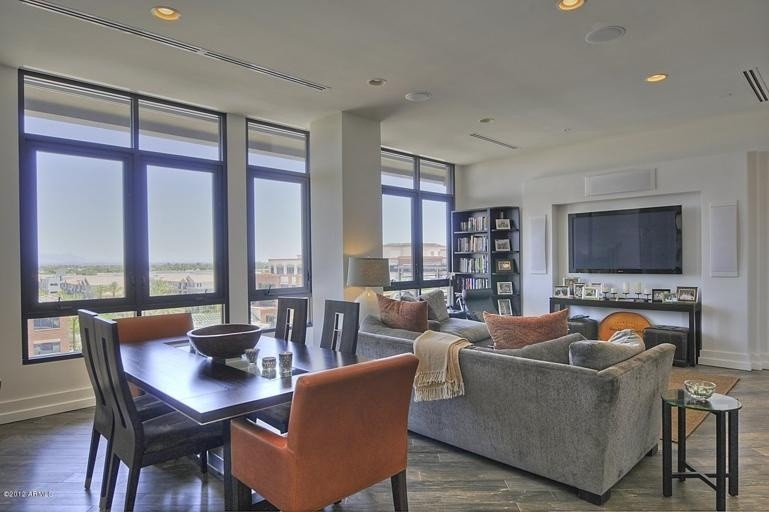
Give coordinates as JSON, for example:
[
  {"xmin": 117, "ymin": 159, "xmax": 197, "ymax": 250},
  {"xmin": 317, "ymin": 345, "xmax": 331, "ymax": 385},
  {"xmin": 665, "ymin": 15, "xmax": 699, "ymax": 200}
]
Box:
[{"xmin": 659, "ymin": 367, "xmax": 741, "ymax": 444}]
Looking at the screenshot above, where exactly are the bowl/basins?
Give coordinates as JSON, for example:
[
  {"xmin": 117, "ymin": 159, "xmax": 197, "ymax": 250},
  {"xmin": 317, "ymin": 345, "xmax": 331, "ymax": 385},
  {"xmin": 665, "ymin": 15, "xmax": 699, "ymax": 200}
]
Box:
[
  {"xmin": 185, "ymin": 321, "xmax": 263, "ymax": 360},
  {"xmin": 684, "ymin": 378, "xmax": 716, "ymax": 401}
]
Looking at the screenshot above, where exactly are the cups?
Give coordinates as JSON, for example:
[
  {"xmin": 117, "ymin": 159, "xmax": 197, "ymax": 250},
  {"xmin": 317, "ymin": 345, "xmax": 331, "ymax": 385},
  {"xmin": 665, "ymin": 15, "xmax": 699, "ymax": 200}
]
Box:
[
  {"xmin": 260, "ymin": 356, "xmax": 276, "ymax": 379},
  {"xmin": 244, "ymin": 348, "xmax": 260, "ymax": 364},
  {"xmin": 279, "ymin": 351, "xmax": 293, "ymax": 373}
]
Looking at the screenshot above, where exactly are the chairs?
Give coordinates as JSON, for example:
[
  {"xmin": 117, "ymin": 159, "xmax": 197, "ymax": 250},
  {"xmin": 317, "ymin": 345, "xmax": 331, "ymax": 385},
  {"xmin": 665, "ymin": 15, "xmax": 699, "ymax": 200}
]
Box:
[
  {"xmin": 257, "ymin": 300, "xmax": 359, "ymax": 435},
  {"xmin": 113, "ymin": 313, "xmax": 193, "ymax": 343},
  {"xmin": 462, "ymin": 287, "xmax": 499, "ymax": 322},
  {"xmin": 76, "ymin": 309, "xmax": 171, "ymax": 509},
  {"xmin": 272, "ymin": 297, "xmax": 307, "ymax": 343},
  {"xmin": 93, "ymin": 313, "xmax": 223, "ymax": 512}
]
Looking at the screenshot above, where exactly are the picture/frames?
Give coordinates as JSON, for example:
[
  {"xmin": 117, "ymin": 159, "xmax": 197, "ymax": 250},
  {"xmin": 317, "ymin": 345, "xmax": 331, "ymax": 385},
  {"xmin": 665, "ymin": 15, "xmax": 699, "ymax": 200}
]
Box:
[
  {"xmin": 498, "ymin": 299, "xmax": 512, "ymax": 316},
  {"xmin": 562, "ymin": 277, "xmax": 580, "ymax": 298},
  {"xmin": 591, "ymin": 282, "xmax": 603, "ymax": 298},
  {"xmin": 651, "ymin": 288, "xmax": 671, "ymax": 303},
  {"xmin": 677, "ymin": 287, "xmax": 698, "ymax": 302},
  {"xmin": 495, "ymin": 239, "xmax": 511, "ymax": 252},
  {"xmin": 581, "ymin": 286, "xmax": 599, "ymax": 301},
  {"xmin": 554, "ymin": 285, "xmax": 570, "ymax": 298},
  {"xmin": 497, "ymin": 281, "xmax": 513, "ymax": 295},
  {"xmin": 661, "ymin": 293, "xmax": 676, "ymax": 304},
  {"xmin": 496, "ymin": 218, "xmax": 511, "ymax": 230},
  {"xmin": 573, "ymin": 283, "xmax": 585, "ymax": 299},
  {"xmin": 494, "ymin": 258, "xmax": 514, "ymax": 273}
]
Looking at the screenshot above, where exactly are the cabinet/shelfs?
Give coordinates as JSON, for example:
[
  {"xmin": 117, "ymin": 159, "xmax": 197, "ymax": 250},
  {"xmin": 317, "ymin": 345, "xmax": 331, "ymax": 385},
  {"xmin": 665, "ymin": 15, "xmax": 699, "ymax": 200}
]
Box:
[
  {"xmin": 550, "ymin": 297, "xmax": 703, "ymax": 368},
  {"xmin": 488, "ymin": 207, "xmax": 523, "ymax": 316},
  {"xmin": 451, "ymin": 207, "xmax": 488, "ymax": 294}
]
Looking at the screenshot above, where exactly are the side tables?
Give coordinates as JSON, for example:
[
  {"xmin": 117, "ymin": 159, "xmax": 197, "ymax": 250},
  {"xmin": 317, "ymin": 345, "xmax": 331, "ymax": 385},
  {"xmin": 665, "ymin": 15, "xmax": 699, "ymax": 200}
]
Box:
[{"xmin": 661, "ymin": 389, "xmax": 742, "ymax": 512}]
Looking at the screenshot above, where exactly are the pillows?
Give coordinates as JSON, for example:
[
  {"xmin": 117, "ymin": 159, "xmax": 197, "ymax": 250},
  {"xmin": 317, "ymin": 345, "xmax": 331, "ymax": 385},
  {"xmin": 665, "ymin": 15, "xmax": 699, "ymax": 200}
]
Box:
[
  {"xmin": 482, "ymin": 307, "xmax": 569, "ymax": 349},
  {"xmin": 400, "ymin": 295, "xmax": 416, "ymax": 305},
  {"xmin": 375, "ymin": 294, "xmax": 428, "ymax": 333},
  {"xmin": 441, "ymin": 316, "xmax": 491, "ymax": 343},
  {"xmin": 567, "ymin": 330, "xmax": 645, "ymax": 370},
  {"xmin": 521, "ymin": 332, "xmax": 588, "ymax": 364},
  {"xmin": 420, "ymin": 289, "xmax": 450, "ymax": 319}
]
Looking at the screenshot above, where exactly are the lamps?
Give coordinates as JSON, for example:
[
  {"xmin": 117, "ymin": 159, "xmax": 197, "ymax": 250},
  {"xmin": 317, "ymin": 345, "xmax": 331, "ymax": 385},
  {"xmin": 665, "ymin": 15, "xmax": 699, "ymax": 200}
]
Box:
[{"xmin": 346, "ymin": 256, "xmax": 391, "ymax": 325}]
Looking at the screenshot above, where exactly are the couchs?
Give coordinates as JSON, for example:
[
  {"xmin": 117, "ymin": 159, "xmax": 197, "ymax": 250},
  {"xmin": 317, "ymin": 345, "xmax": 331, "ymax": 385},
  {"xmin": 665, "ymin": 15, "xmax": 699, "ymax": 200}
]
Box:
[
  {"xmin": 355, "ymin": 327, "xmax": 676, "ymax": 505},
  {"xmin": 230, "ymin": 352, "xmax": 419, "ymax": 512}
]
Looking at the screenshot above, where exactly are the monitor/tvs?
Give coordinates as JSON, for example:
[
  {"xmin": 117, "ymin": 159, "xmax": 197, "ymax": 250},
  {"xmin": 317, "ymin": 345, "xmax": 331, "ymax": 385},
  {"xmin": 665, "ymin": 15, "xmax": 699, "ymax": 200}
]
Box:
[{"xmin": 568, "ymin": 204, "xmax": 683, "ymax": 275}]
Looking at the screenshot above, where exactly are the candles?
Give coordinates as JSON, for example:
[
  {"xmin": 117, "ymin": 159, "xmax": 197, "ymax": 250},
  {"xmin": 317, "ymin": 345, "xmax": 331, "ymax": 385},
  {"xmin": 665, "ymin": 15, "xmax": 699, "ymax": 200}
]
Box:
[
  {"xmin": 644, "ymin": 288, "xmax": 650, "ymax": 294},
  {"xmin": 603, "ymin": 286, "xmax": 608, "ymax": 291},
  {"xmin": 635, "ymin": 280, "xmax": 641, "ymax": 293},
  {"xmin": 622, "ymin": 281, "xmax": 629, "ymax": 292},
  {"xmin": 611, "ymin": 288, "xmax": 617, "ymax": 292}
]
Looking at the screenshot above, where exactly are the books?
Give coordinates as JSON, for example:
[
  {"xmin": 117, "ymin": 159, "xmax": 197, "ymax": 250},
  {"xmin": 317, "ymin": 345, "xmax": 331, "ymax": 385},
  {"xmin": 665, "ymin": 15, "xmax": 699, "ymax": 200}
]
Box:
[
  {"xmin": 459, "ymin": 216, "xmax": 487, "ymax": 231},
  {"xmin": 463, "ymin": 277, "xmax": 488, "ymax": 289},
  {"xmin": 457, "ymin": 234, "xmax": 488, "ymax": 253},
  {"xmin": 457, "ymin": 254, "xmax": 487, "ymax": 273}
]
